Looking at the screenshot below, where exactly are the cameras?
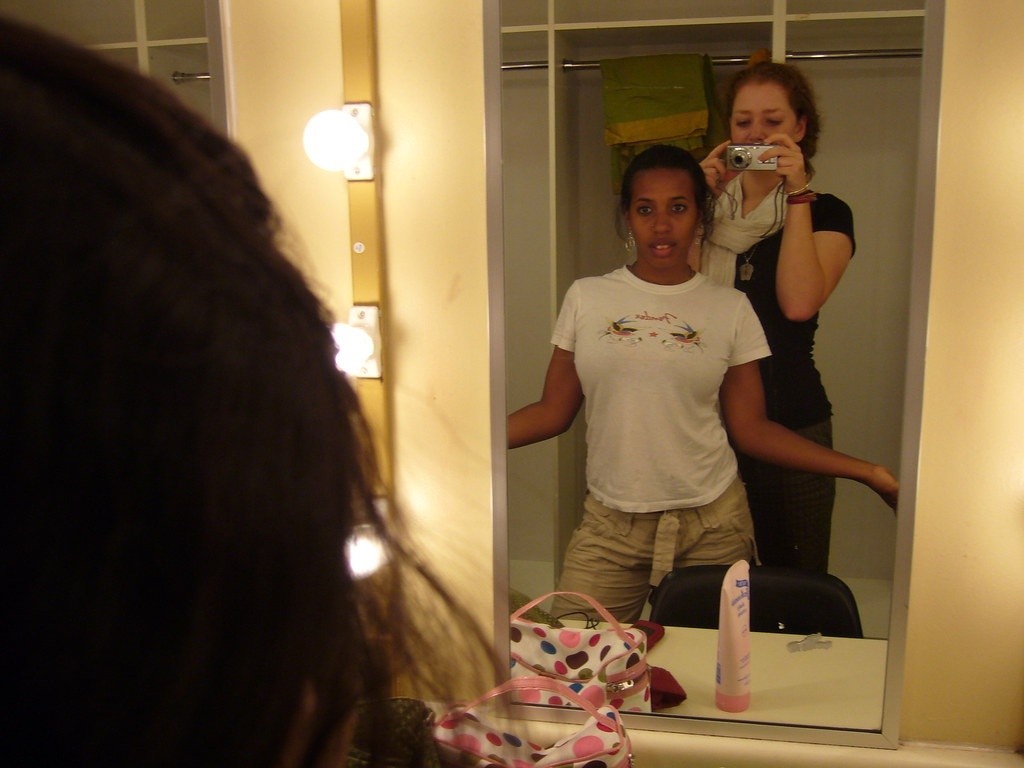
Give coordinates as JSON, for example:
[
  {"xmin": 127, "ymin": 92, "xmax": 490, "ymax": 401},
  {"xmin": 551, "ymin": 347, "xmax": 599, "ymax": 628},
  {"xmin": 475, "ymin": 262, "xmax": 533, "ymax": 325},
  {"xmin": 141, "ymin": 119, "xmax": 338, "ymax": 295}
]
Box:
[{"xmin": 726, "ymin": 144, "xmax": 778, "ymax": 171}]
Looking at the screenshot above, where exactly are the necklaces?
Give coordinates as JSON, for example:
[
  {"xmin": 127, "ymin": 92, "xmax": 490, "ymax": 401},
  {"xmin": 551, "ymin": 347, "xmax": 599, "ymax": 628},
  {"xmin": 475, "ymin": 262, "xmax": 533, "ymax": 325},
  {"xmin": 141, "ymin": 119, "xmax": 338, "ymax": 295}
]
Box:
[{"xmin": 741, "ymin": 245, "xmax": 757, "ymax": 281}]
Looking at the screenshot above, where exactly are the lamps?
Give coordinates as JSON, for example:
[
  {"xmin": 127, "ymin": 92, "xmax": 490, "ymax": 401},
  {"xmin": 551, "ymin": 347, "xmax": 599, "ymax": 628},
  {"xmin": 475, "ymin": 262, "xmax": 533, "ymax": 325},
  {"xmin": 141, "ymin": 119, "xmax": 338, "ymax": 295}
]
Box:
[
  {"xmin": 303, "ymin": 102, "xmax": 377, "ymax": 181},
  {"xmin": 332, "ymin": 304, "xmax": 384, "ymax": 379},
  {"xmin": 345, "ymin": 497, "xmax": 396, "ymax": 576}
]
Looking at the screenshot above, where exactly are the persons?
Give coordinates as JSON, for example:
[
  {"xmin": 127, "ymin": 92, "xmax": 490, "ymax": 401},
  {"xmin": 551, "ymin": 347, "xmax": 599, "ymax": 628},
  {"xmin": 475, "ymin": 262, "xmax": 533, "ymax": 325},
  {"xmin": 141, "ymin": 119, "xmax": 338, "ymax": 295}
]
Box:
[
  {"xmin": 506, "ymin": 147, "xmax": 899, "ymax": 625},
  {"xmin": 688, "ymin": 64, "xmax": 857, "ymax": 572},
  {"xmin": 1, "ymin": 17, "xmax": 484, "ymax": 768}
]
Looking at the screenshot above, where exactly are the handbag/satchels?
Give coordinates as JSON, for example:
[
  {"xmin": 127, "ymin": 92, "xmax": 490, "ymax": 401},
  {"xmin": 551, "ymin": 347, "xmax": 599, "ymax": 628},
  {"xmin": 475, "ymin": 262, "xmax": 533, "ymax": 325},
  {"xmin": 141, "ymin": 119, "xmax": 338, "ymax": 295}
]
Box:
[
  {"xmin": 431, "ymin": 676, "xmax": 636, "ymax": 767},
  {"xmin": 505, "ymin": 589, "xmax": 652, "ymax": 712}
]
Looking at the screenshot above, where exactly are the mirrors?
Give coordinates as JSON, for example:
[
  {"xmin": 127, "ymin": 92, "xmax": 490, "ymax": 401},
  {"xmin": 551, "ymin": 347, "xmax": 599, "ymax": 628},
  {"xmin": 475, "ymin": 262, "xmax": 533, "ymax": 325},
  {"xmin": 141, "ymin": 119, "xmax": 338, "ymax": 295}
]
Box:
[{"xmin": 481, "ymin": 0, "xmax": 947, "ymax": 748}]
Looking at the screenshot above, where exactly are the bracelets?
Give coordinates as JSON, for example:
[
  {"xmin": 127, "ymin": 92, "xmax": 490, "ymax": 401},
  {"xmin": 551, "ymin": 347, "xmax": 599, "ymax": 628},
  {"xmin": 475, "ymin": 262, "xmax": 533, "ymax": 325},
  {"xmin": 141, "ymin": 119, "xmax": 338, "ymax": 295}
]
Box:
[{"xmin": 787, "ymin": 182, "xmax": 817, "ymax": 204}]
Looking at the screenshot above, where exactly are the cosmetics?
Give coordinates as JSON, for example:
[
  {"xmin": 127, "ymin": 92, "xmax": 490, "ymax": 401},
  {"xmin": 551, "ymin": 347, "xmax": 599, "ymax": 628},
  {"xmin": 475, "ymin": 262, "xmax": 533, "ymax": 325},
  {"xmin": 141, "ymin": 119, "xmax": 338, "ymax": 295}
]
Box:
[{"xmin": 716, "ymin": 560, "xmax": 752, "ymax": 713}]
